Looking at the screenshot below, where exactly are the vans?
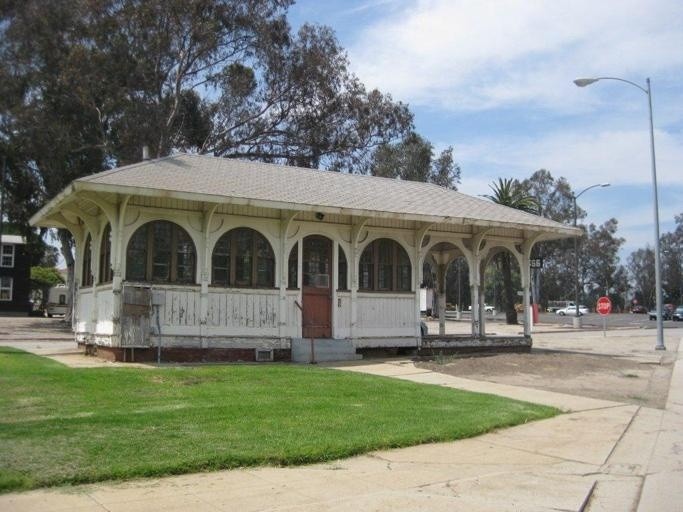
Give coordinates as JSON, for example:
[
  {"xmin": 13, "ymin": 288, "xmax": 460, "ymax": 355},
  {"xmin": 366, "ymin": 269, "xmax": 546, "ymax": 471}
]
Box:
[{"xmin": 556, "ymin": 305, "xmax": 589, "ymax": 317}]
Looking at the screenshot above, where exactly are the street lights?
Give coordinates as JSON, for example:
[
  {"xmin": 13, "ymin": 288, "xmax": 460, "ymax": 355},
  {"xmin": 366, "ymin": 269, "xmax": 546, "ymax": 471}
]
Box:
[
  {"xmin": 573, "ymin": 77, "xmax": 667, "ymax": 351},
  {"xmin": 568, "ymin": 182, "xmax": 609, "ymax": 332}
]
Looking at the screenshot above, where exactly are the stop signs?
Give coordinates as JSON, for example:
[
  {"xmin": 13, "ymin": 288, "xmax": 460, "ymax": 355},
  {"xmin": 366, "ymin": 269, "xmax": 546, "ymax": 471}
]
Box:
[{"xmin": 596, "ymin": 297, "xmax": 612, "ymax": 316}]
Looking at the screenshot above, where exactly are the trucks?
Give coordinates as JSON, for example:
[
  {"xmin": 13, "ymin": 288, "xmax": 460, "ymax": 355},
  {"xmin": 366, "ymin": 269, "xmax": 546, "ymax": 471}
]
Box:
[
  {"xmin": 546, "ymin": 300, "xmax": 576, "ymax": 312},
  {"xmin": 47, "ymin": 284, "xmax": 68, "ymax": 318}
]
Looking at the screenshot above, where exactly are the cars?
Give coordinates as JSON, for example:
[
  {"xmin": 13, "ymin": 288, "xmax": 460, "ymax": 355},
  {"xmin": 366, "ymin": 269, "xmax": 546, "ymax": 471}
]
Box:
[
  {"xmin": 648, "ymin": 306, "xmax": 672, "ymax": 320},
  {"xmin": 671, "ymin": 306, "xmax": 682, "ymax": 321},
  {"xmin": 663, "ymin": 303, "xmax": 676, "ymax": 314},
  {"xmin": 514, "ymin": 305, "xmax": 524, "ymax": 312},
  {"xmin": 632, "ymin": 305, "xmax": 647, "ymax": 314}
]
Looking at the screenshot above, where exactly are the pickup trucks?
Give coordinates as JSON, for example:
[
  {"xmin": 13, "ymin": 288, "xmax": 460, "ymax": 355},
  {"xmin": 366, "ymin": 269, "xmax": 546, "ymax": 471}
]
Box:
[{"xmin": 467, "ymin": 303, "xmax": 496, "ymax": 313}]
